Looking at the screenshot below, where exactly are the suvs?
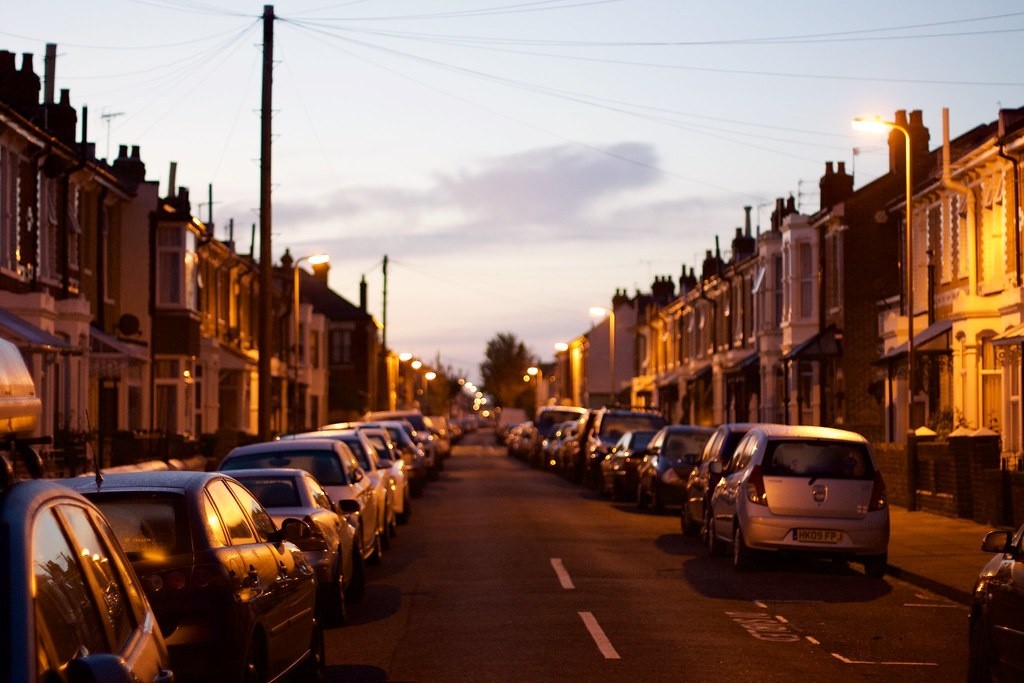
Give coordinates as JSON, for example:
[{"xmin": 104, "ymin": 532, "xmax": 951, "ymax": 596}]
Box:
[{"xmin": 586, "ymin": 406, "xmax": 668, "ymax": 487}]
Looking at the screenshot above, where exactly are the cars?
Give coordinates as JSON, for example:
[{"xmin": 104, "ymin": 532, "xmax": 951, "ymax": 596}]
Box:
[
  {"xmin": 704, "ymin": 425, "xmax": 891, "ymax": 576},
  {"xmin": 51, "ymin": 474, "xmax": 325, "ymax": 683},
  {"xmin": 214, "ymin": 469, "xmax": 365, "ymax": 628},
  {"xmin": 599, "ymin": 429, "xmax": 662, "ymax": 501},
  {"xmin": 677, "ymin": 424, "xmax": 783, "ymax": 537},
  {"xmin": 630, "ymin": 424, "xmax": 716, "ymax": 514},
  {"xmin": 967, "ymin": 521, "xmax": 1024, "ymax": 682},
  {"xmin": 218, "ymin": 438, "xmax": 389, "ymax": 577},
  {"xmin": 0, "ymin": 337, "xmax": 174, "ymax": 683},
  {"xmin": 495, "ymin": 406, "xmax": 593, "ymax": 481},
  {"xmin": 276, "ymin": 408, "xmax": 480, "ymax": 548}
]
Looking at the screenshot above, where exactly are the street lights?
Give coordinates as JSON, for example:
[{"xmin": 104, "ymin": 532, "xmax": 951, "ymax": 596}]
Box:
[
  {"xmin": 289, "ymin": 254, "xmax": 332, "ymax": 436},
  {"xmin": 850, "ymin": 117, "xmax": 919, "ymax": 512},
  {"xmin": 591, "ymin": 306, "xmax": 616, "ymax": 409}
]
